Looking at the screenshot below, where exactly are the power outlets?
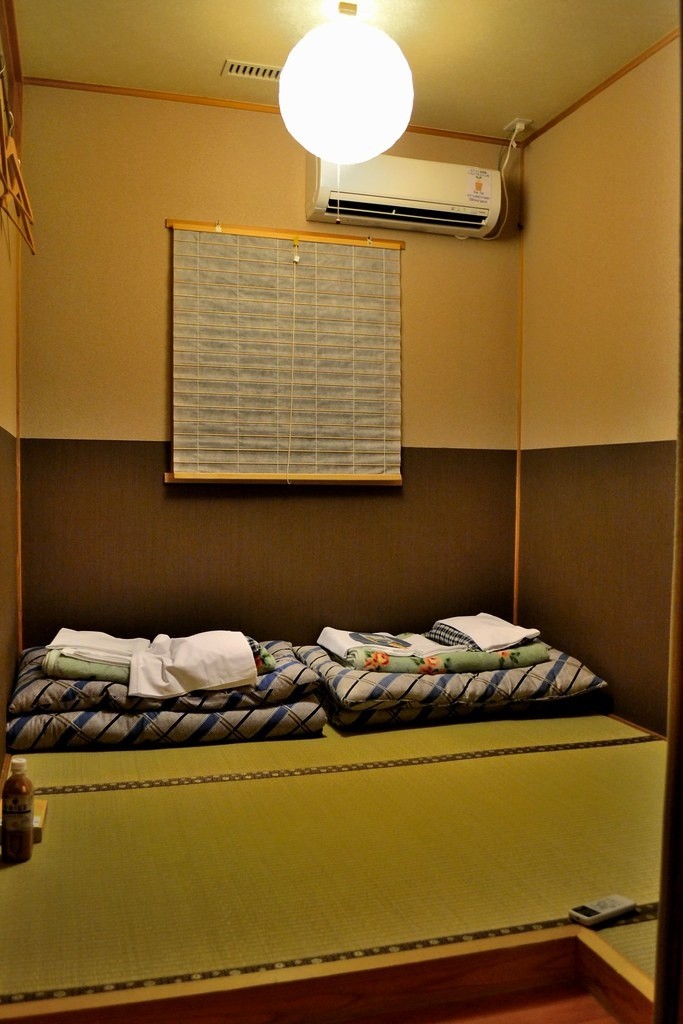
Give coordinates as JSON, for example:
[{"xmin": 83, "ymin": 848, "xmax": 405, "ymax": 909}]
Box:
[{"xmin": 503, "ymin": 118, "xmax": 532, "ymax": 133}]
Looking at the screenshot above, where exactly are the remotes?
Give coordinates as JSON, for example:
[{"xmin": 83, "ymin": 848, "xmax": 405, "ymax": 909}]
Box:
[{"xmin": 568, "ymin": 894, "xmax": 636, "ymax": 926}]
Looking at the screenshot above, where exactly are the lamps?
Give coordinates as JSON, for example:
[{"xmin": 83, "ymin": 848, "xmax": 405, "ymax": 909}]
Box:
[{"xmin": 277, "ymin": 2, "xmax": 415, "ymax": 166}]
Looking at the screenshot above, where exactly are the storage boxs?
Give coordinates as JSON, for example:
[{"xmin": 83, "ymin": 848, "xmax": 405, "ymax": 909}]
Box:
[{"xmin": 0, "ymin": 798, "xmax": 48, "ymax": 842}]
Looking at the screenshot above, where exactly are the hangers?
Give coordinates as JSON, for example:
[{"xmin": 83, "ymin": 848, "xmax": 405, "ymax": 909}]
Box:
[{"xmin": 0, "ymin": 110, "xmax": 36, "ymax": 255}]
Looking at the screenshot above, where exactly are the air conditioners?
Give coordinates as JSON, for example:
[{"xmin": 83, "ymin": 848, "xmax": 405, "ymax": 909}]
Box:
[{"xmin": 308, "ymin": 151, "xmax": 503, "ymax": 238}]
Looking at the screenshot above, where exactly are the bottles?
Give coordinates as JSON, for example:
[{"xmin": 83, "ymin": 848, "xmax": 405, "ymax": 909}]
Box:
[{"xmin": 1, "ymin": 757, "xmax": 34, "ymax": 863}]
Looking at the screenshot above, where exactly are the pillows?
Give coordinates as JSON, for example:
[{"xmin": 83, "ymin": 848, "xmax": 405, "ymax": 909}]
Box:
[{"xmin": 428, "ymin": 623, "xmax": 535, "ymax": 652}]
